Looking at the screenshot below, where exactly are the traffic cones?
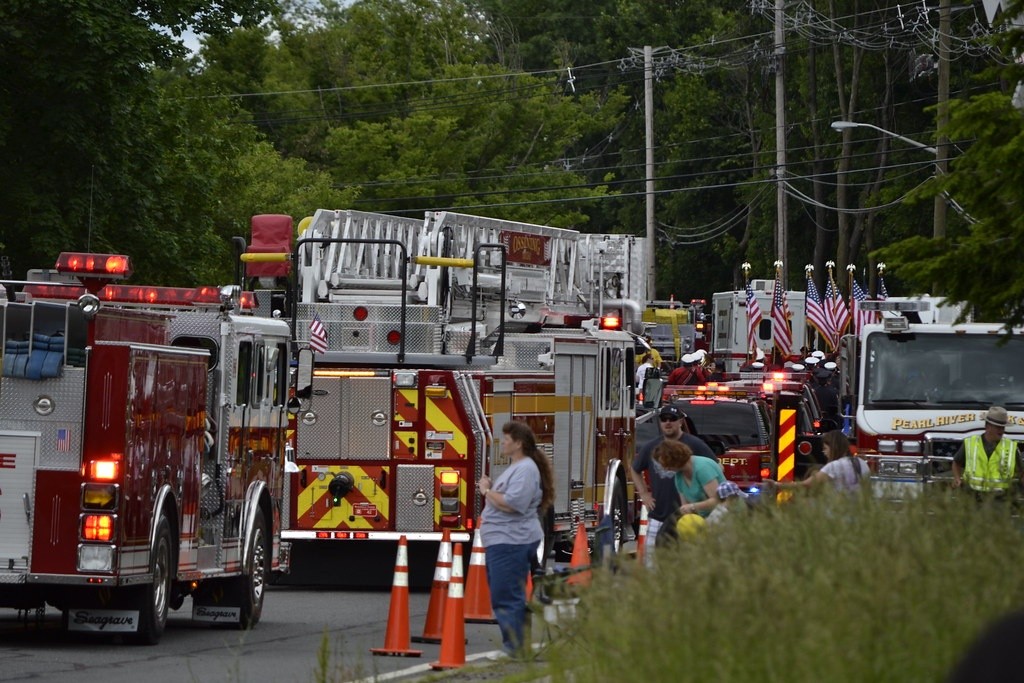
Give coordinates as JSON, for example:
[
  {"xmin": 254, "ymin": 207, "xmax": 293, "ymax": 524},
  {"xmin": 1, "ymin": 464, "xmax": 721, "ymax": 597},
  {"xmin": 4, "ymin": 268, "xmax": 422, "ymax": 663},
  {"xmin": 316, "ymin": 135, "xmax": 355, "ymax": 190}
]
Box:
[
  {"xmin": 411, "ymin": 528, "xmax": 468, "ymax": 645},
  {"xmin": 369, "ymin": 534, "xmax": 423, "ymax": 657},
  {"xmin": 636, "ymin": 504, "xmax": 649, "ymax": 565},
  {"xmin": 562, "ymin": 521, "xmax": 592, "ymax": 595},
  {"xmin": 464, "ymin": 516, "xmax": 500, "ymax": 624},
  {"xmin": 429, "ymin": 542, "xmax": 467, "ymax": 669},
  {"xmin": 524, "ymin": 567, "xmax": 534, "ymax": 608}
]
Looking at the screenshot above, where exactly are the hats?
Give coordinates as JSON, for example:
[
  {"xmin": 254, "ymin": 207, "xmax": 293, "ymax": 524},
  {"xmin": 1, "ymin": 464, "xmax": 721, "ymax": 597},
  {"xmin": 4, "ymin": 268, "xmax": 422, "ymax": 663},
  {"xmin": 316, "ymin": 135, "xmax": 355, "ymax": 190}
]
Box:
[
  {"xmin": 755, "ymin": 355, "xmax": 765, "ymax": 362},
  {"xmin": 791, "ymin": 364, "xmax": 805, "ymax": 371},
  {"xmin": 681, "ymin": 354, "xmax": 694, "ymax": 362},
  {"xmin": 654, "ymin": 405, "xmax": 687, "ymax": 420},
  {"xmin": 676, "ymin": 514, "xmax": 705, "ymax": 541},
  {"xmin": 716, "ymin": 481, "xmax": 748, "ymax": 499},
  {"xmin": 752, "ymin": 362, "xmax": 765, "ymax": 369},
  {"xmin": 811, "ymin": 350, "xmax": 824, "ymax": 357},
  {"xmin": 696, "ymin": 348, "xmax": 706, "ymax": 358},
  {"xmin": 691, "ymin": 353, "xmax": 702, "ymax": 361},
  {"xmin": 981, "ymin": 407, "xmax": 1014, "ymax": 427},
  {"xmin": 805, "ymin": 356, "xmax": 819, "ymax": 366},
  {"xmin": 824, "ymin": 362, "xmax": 837, "ymax": 371}
]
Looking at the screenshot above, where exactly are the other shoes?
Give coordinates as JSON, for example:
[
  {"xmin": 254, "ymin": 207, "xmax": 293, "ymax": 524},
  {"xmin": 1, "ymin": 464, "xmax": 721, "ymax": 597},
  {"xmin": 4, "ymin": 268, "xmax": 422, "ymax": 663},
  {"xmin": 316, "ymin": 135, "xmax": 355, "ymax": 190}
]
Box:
[{"xmin": 485, "ymin": 649, "xmax": 522, "ymax": 662}]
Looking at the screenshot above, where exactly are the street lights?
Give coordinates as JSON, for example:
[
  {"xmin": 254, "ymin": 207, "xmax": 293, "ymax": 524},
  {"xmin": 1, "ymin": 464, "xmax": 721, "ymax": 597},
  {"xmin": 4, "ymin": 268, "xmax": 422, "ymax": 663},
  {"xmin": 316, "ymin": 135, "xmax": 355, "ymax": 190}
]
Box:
[{"xmin": 832, "ymin": 120, "xmax": 946, "ymax": 296}]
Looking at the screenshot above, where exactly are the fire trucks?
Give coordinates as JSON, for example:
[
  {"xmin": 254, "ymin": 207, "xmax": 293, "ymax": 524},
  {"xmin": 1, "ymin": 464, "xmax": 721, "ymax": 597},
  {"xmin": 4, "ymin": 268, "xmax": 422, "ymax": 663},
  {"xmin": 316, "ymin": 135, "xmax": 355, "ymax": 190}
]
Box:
[
  {"xmin": 0, "ymin": 251, "xmax": 315, "ymax": 644},
  {"xmin": 230, "ymin": 209, "xmax": 666, "ymax": 588}
]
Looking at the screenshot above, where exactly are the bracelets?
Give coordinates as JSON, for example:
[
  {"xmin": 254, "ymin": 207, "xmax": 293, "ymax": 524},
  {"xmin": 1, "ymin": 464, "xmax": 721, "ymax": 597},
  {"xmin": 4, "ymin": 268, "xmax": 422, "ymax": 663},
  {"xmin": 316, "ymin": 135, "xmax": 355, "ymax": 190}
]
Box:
[
  {"xmin": 483, "ymin": 488, "xmax": 490, "ymax": 496},
  {"xmin": 773, "ymin": 481, "xmax": 777, "ymax": 488},
  {"xmin": 690, "ymin": 503, "xmax": 696, "ymax": 513}
]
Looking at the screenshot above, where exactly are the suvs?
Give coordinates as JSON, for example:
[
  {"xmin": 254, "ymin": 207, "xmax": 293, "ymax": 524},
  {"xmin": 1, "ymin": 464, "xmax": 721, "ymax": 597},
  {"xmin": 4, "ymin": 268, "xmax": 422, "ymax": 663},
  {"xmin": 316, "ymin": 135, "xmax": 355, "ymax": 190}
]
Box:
[{"xmin": 635, "ymin": 370, "xmax": 838, "ymax": 517}]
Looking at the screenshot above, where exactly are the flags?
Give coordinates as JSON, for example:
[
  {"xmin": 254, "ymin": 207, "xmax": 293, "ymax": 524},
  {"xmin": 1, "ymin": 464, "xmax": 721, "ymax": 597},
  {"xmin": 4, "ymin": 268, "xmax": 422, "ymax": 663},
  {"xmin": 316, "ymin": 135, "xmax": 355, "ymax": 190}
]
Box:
[
  {"xmin": 876, "ymin": 276, "xmax": 888, "ymax": 301},
  {"xmin": 805, "ymin": 278, "xmax": 836, "ymax": 351},
  {"xmin": 823, "ymin": 277, "xmax": 852, "ymax": 348},
  {"xmin": 308, "ymin": 312, "xmax": 327, "ymax": 355},
  {"xmin": 744, "ymin": 279, "xmax": 763, "ymax": 350},
  {"xmin": 852, "ymin": 278, "xmax": 880, "ymax": 342},
  {"xmin": 770, "ymin": 274, "xmax": 794, "ymax": 358}
]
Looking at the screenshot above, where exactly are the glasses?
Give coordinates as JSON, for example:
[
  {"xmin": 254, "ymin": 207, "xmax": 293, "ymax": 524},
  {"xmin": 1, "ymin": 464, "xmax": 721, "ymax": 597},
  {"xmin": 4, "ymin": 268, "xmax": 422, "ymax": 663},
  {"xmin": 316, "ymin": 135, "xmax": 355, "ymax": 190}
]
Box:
[{"xmin": 657, "ymin": 415, "xmax": 677, "ymax": 423}]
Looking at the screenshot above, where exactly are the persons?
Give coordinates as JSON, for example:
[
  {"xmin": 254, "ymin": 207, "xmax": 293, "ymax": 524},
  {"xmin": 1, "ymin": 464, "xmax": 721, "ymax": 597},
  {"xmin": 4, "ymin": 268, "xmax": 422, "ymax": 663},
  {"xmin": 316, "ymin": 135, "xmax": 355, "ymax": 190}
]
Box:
[
  {"xmin": 755, "ymin": 427, "xmax": 872, "ymax": 501},
  {"xmin": 635, "ymin": 334, "xmax": 663, "ymax": 409},
  {"xmin": 896, "ymin": 349, "xmax": 944, "ymax": 401},
  {"xmin": 952, "ymin": 352, "xmax": 994, "ymax": 389},
  {"xmin": 667, "ymin": 348, "xmax": 735, "ymax": 386},
  {"xmin": 740, "ymin": 345, "xmax": 841, "ymax": 419},
  {"xmin": 951, "ymin": 406, "xmax": 1024, "ymax": 508},
  {"xmin": 650, "ymin": 441, "xmax": 728, "ymax": 519},
  {"xmin": 630, "ymin": 404, "xmax": 724, "ymax": 572},
  {"xmin": 478, "ymin": 422, "xmax": 556, "ymax": 663}
]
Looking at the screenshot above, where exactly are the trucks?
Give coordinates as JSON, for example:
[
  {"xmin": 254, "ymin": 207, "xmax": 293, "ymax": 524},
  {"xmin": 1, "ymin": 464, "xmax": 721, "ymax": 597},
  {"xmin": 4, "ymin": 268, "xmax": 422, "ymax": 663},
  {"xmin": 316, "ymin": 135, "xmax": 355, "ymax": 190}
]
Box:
[
  {"xmin": 841, "ymin": 292, "xmax": 1024, "ymax": 524},
  {"xmin": 705, "ymin": 279, "xmax": 818, "ymax": 381},
  {"xmin": 639, "ymin": 298, "xmax": 713, "ymax": 386}
]
